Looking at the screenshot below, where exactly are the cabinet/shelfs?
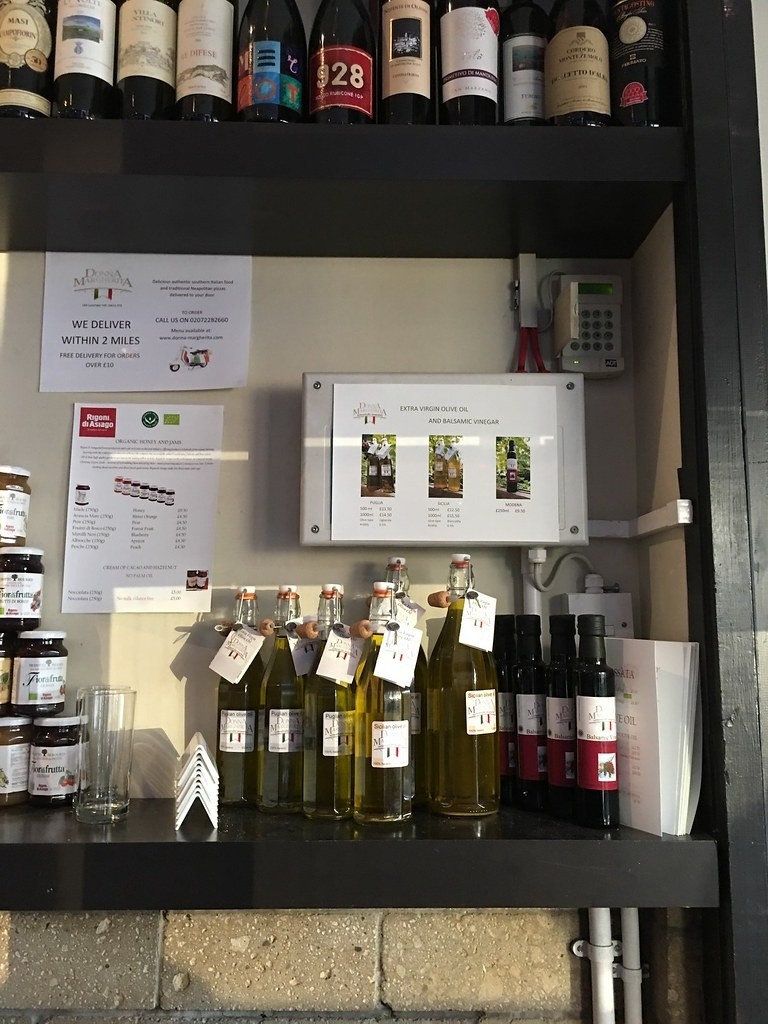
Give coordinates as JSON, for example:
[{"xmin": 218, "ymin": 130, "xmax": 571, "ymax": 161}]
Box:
[{"xmin": 0, "ymin": 0, "xmax": 768, "ymax": 1023}]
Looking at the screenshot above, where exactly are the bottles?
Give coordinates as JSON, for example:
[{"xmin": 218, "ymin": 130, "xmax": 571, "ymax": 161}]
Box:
[
  {"xmin": 255, "ymin": 554, "xmax": 618, "ymax": 831},
  {"xmin": 378, "ymin": 2, "xmax": 435, "ymax": 125},
  {"xmin": 218, "ymin": 586, "xmax": 266, "ymax": 810},
  {"xmin": 53, "ymin": 1, "xmax": 118, "ymax": 120},
  {"xmin": 307, "ymin": 1, "xmax": 376, "ymax": 125},
  {"xmin": 236, "ymin": 1, "xmax": 305, "ymax": 123},
  {"xmin": 117, "ymin": 1, "xmax": 175, "ymax": 118},
  {"xmin": 1, "ymin": 1, "xmax": 51, "ymax": 119},
  {"xmin": 0, "ymin": 466, "xmax": 92, "ymax": 810},
  {"xmin": 175, "ymin": 2, "xmax": 235, "ymax": 121},
  {"xmin": 437, "ymin": 0, "xmax": 671, "ymax": 127}
]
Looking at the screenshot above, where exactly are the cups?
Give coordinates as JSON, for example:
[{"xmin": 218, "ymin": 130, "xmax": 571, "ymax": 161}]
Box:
[{"xmin": 74, "ymin": 686, "xmax": 137, "ymax": 826}]
